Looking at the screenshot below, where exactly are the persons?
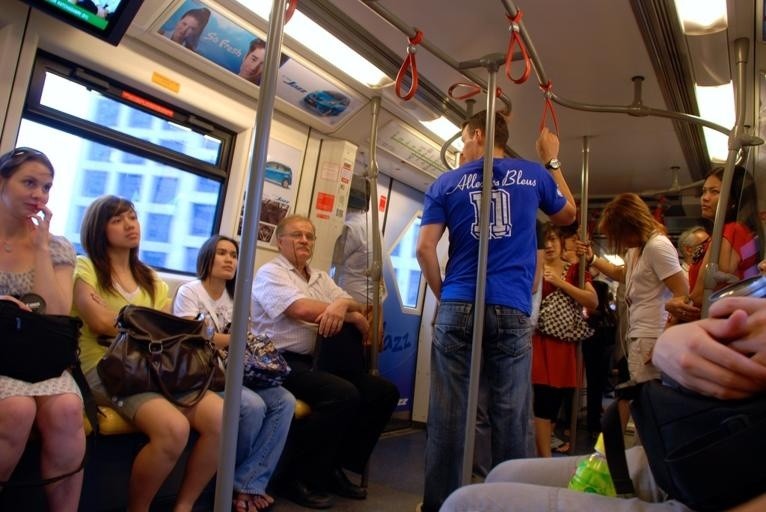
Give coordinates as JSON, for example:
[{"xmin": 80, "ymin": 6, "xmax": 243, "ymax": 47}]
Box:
[
  {"xmin": 331, "ymin": 174, "xmax": 388, "ymax": 354},
  {"xmin": 528, "ymin": 166, "xmax": 766, "ymax": 455},
  {"xmin": 250, "ymin": 214, "xmax": 401, "ymax": 510},
  {"xmin": 173, "ymin": 235, "xmax": 296, "ymax": 512},
  {"xmin": 70, "ymin": 195, "xmax": 224, "ymax": 512},
  {"xmin": 439, "ymin": 259, "xmax": 766, "ymax": 512},
  {"xmin": 0, "ymin": 146, "xmax": 86, "ymax": 512},
  {"xmin": 238, "ymin": 39, "xmax": 289, "ymax": 86},
  {"xmin": 158, "ymin": 8, "xmax": 211, "ymax": 53},
  {"xmin": 417, "ymin": 110, "xmax": 576, "ymax": 512}
]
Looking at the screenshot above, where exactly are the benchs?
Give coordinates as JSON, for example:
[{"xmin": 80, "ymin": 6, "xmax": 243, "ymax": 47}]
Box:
[{"xmin": 85, "ymin": 269, "xmax": 311, "ymax": 439}]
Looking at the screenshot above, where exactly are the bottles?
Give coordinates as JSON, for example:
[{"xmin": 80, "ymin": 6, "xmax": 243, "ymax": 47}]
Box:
[
  {"xmin": 204, "ymin": 316, "xmax": 214, "ymax": 342},
  {"xmin": 566, "ymin": 431, "xmax": 619, "ymax": 499}
]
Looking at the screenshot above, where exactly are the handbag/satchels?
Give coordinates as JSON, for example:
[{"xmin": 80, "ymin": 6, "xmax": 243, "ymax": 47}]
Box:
[
  {"xmin": 0, "ymin": 300, "xmax": 82, "ymax": 384},
  {"xmin": 628, "ymin": 353, "xmax": 766, "ymax": 512},
  {"xmin": 223, "ymin": 331, "xmax": 292, "ymax": 387},
  {"xmin": 537, "ymin": 291, "xmax": 596, "ymax": 342},
  {"xmin": 97, "ymin": 304, "xmax": 225, "ymax": 396}
]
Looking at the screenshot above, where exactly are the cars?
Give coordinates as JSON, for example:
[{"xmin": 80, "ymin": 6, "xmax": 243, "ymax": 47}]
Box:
[
  {"xmin": 264, "ymin": 162, "xmax": 293, "ymax": 188},
  {"xmin": 304, "ymin": 90, "xmax": 350, "ymax": 117}
]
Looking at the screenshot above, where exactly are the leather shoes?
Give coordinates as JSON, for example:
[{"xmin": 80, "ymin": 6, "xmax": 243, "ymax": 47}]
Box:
[
  {"xmin": 334, "ymin": 472, "xmax": 367, "ymax": 499},
  {"xmin": 295, "ymin": 481, "xmax": 335, "ymax": 508}
]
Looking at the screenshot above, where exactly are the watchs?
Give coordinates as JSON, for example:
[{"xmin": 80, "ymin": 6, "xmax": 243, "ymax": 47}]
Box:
[{"xmin": 545, "ymin": 159, "xmax": 561, "ymax": 170}]
